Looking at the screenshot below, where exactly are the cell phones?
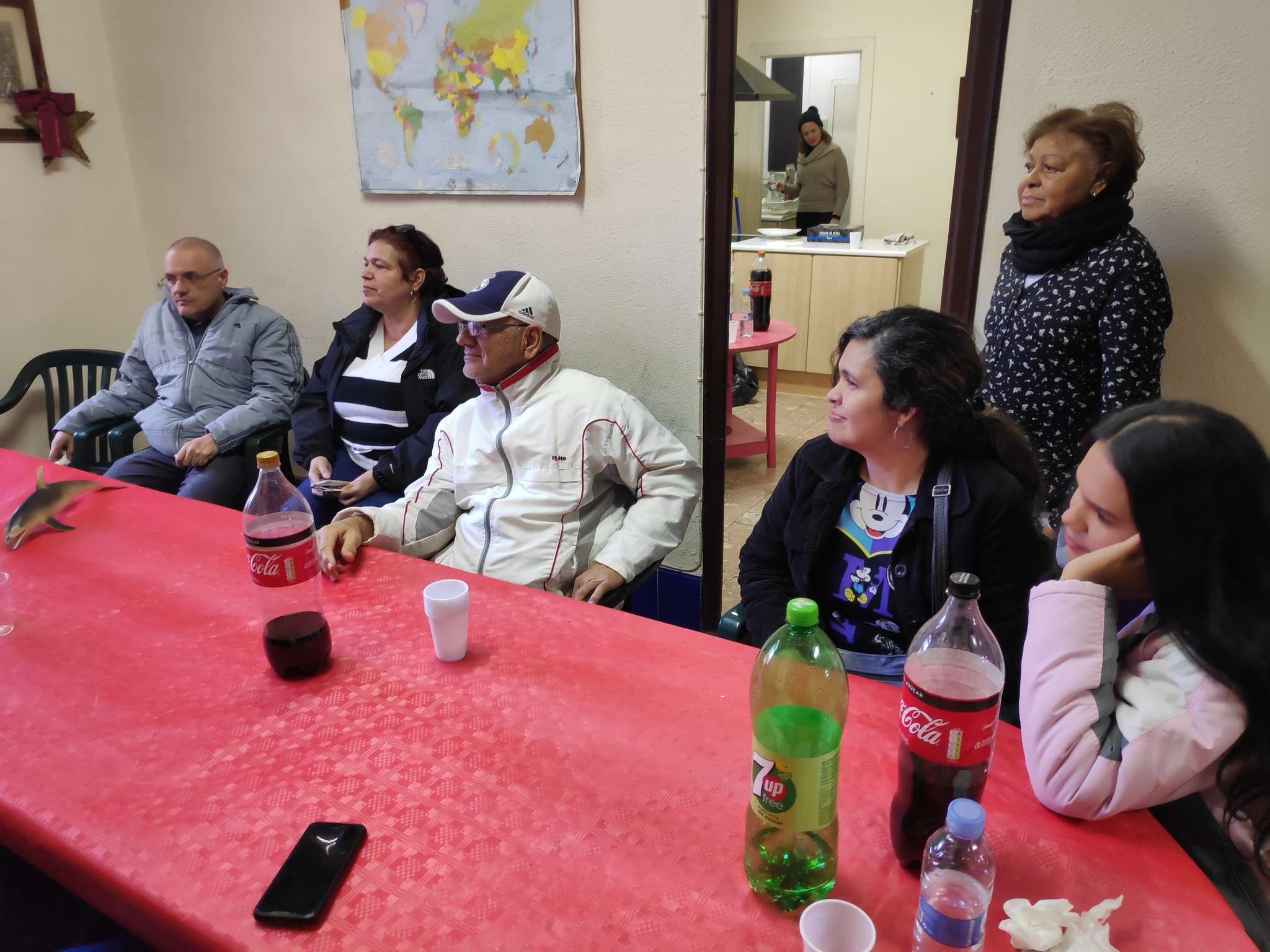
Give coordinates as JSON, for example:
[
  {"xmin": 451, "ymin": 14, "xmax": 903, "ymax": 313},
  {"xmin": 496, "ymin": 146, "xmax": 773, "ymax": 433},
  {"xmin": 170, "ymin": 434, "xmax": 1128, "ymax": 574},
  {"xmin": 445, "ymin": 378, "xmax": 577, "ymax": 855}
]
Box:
[
  {"xmin": 310, "ymin": 479, "xmax": 353, "ymax": 490},
  {"xmin": 253, "ymin": 821, "xmax": 368, "ymax": 928}
]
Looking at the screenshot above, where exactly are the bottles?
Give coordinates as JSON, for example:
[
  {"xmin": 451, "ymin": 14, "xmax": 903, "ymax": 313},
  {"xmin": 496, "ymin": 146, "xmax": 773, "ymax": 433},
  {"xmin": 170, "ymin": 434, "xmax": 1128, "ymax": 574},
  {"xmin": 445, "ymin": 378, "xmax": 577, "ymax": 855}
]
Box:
[
  {"xmin": 743, "ymin": 597, "xmax": 851, "ymax": 911},
  {"xmin": 729, "ymin": 290, "xmax": 735, "ymax": 323},
  {"xmin": 739, "ymin": 289, "xmax": 753, "ymax": 338},
  {"xmin": 749, "ymin": 250, "xmax": 772, "ymax": 332},
  {"xmin": 242, "ymin": 451, "xmax": 332, "ymax": 679},
  {"xmin": 888, "ymin": 571, "xmax": 1006, "ymax": 867},
  {"xmin": 911, "ymin": 797, "xmax": 997, "ymax": 952},
  {"xmin": 769, "ymin": 170, "xmax": 781, "ymax": 203}
]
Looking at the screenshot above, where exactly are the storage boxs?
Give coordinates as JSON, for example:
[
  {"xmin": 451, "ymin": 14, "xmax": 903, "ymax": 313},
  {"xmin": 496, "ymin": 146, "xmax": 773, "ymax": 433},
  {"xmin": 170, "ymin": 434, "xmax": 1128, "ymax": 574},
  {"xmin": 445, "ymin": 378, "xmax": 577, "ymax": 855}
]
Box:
[{"xmin": 807, "ymin": 224, "xmax": 865, "ymax": 242}]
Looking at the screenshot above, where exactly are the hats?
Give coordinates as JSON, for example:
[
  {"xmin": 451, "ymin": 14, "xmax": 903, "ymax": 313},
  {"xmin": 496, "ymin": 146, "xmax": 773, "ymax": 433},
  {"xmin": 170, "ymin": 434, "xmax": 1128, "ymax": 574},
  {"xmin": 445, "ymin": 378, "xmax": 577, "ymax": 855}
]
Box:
[{"xmin": 432, "ymin": 272, "xmax": 560, "ymax": 341}]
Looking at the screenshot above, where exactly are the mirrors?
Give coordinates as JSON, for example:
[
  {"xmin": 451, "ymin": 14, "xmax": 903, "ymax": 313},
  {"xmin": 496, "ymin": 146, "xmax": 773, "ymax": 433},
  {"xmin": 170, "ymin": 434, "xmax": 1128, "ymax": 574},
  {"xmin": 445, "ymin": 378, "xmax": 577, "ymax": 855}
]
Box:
[{"xmin": 753, "ymin": 36, "xmax": 875, "ymax": 236}]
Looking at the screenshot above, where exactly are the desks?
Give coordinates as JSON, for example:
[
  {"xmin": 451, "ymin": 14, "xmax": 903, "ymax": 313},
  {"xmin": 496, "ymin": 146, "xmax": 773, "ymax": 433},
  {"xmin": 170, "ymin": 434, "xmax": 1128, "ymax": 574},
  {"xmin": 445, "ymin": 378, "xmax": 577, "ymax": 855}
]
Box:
[
  {"xmin": 0, "ymin": 448, "xmax": 1261, "ymax": 952},
  {"xmin": 726, "ymin": 313, "xmax": 797, "ymax": 468}
]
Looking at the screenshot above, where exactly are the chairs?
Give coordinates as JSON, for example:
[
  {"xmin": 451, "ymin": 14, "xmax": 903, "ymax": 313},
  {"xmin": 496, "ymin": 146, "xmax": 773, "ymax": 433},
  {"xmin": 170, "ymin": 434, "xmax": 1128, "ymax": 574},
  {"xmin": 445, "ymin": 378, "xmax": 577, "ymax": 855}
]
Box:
[{"xmin": 0, "ymin": 349, "xmax": 310, "ymax": 513}]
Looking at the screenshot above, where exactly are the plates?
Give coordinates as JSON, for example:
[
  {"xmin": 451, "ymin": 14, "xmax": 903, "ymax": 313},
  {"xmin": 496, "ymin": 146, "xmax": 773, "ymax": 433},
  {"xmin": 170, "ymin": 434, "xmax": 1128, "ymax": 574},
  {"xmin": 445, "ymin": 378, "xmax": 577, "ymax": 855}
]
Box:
[{"xmin": 757, "ymin": 228, "xmax": 802, "ymax": 237}]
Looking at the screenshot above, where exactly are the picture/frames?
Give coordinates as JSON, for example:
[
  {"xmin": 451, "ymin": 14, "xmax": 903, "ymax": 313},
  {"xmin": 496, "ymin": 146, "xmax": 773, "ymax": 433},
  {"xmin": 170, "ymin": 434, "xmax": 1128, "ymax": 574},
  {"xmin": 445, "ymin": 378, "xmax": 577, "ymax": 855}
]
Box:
[{"xmin": 0, "ymin": 0, "xmax": 52, "ymax": 141}]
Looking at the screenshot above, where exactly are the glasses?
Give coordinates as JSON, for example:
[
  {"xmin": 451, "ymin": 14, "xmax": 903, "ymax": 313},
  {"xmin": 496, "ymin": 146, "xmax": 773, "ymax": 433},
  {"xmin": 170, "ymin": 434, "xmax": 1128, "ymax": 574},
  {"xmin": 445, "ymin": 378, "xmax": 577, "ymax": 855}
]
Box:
[
  {"xmin": 161, "ymin": 267, "xmax": 223, "ymax": 287},
  {"xmin": 456, "ymin": 321, "xmax": 530, "ymax": 335}
]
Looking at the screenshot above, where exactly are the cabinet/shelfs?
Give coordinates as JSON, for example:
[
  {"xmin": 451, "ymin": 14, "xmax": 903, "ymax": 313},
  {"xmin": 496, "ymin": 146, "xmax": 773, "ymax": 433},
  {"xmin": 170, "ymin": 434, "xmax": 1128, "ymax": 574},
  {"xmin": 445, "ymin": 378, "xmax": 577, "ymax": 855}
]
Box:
[{"xmin": 731, "ymin": 251, "xmax": 925, "ymax": 387}]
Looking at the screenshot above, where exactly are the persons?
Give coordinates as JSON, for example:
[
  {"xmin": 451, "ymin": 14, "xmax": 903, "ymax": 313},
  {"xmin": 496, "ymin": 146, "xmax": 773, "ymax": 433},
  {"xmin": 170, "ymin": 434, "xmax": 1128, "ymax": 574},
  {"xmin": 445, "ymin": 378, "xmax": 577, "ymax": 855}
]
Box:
[
  {"xmin": 48, "ymin": 236, "xmax": 304, "ymax": 508},
  {"xmin": 279, "ymin": 225, "xmax": 480, "ymax": 530},
  {"xmin": 775, "ymin": 106, "xmax": 850, "ymax": 235},
  {"xmin": 976, "ymin": 101, "xmax": 1171, "ymax": 532},
  {"xmin": 736, "ymin": 306, "xmax": 1055, "ymax": 726},
  {"xmin": 1018, "ymin": 399, "xmax": 1270, "ymax": 952},
  {"xmin": 314, "ymin": 271, "xmax": 703, "ymax": 604}
]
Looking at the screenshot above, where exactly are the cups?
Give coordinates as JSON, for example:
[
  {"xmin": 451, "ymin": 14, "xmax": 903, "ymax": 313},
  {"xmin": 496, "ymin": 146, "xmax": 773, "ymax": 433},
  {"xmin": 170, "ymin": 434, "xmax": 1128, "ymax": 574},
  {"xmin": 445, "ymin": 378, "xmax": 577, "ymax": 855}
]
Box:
[
  {"xmin": 0, "ymin": 572, "xmax": 15, "ymax": 636},
  {"xmin": 729, "ymin": 321, "xmax": 739, "ymax": 343},
  {"xmin": 424, "ymin": 579, "xmax": 469, "ymax": 662},
  {"xmin": 849, "ymin": 232, "xmax": 862, "ymax": 248},
  {"xmin": 799, "ymin": 898, "xmax": 877, "ymax": 952}
]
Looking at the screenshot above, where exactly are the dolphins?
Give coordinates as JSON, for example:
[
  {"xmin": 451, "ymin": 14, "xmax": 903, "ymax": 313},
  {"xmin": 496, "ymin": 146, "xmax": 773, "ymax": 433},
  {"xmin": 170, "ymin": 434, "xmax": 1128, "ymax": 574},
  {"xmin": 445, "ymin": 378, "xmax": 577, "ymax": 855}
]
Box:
[{"xmin": 4, "ymin": 465, "xmax": 128, "ymax": 550}]
